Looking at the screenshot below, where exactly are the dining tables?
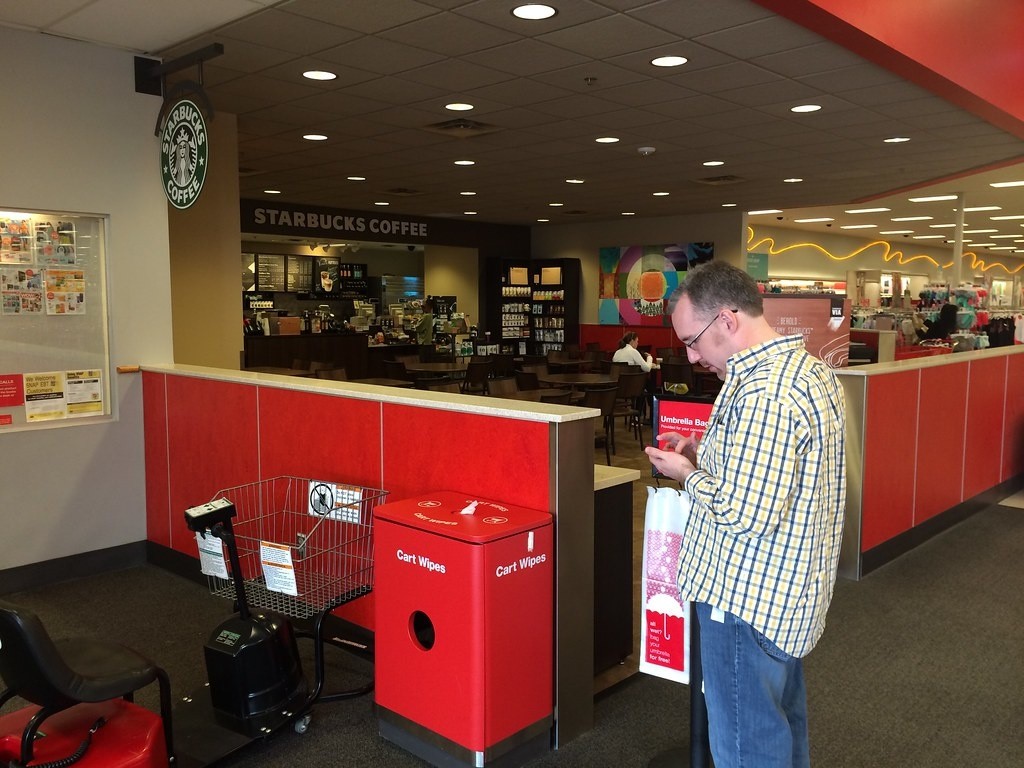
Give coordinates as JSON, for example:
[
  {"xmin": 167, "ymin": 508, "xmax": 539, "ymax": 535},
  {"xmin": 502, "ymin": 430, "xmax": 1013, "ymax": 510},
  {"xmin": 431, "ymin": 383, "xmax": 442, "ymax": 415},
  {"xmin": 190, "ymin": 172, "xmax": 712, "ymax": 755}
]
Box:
[
  {"xmin": 490, "ymin": 389, "xmax": 586, "ymax": 402},
  {"xmin": 243, "ymin": 366, "xmax": 313, "ymax": 375},
  {"xmin": 349, "ymin": 378, "xmax": 415, "ymax": 386},
  {"xmin": 405, "ymin": 363, "xmax": 467, "ymax": 383}
]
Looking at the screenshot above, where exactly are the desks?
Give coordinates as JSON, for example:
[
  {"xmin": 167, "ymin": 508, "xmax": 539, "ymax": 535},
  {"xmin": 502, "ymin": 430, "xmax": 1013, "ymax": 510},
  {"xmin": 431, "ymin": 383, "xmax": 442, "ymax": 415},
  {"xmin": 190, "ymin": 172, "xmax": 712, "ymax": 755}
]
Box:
[
  {"xmin": 538, "ymin": 374, "xmax": 619, "ymax": 387},
  {"xmin": 693, "ymin": 365, "xmax": 713, "ymax": 373},
  {"xmin": 549, "ymin": 361, "xmax": 592, "ymax": 373}
]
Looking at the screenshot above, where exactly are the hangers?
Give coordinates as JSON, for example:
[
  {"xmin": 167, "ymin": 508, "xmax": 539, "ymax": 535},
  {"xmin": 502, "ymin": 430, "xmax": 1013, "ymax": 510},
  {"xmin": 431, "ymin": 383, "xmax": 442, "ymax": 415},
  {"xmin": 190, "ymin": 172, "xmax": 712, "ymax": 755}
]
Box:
[
  {"xmin": 989, "ymin": 312, "xmax": 1022, "ymax": 318},
  {"xmin": 780, "ymin": 287, "xmax": 833, "ymax": 294},
  {"xmin": 923, "ymin": 284, "xmax": 946, "ymax": 291},
  {"xmin": 951, "ymin": 330, "xmax": 988, "ymax": 338}
]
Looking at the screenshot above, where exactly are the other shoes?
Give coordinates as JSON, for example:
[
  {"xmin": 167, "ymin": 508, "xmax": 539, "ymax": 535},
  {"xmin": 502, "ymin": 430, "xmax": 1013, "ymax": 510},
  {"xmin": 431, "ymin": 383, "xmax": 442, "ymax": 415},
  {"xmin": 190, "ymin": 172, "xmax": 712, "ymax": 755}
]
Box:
[{"xmin": 631, "ymin": 420, "xmax": 644, "ymax": 426}]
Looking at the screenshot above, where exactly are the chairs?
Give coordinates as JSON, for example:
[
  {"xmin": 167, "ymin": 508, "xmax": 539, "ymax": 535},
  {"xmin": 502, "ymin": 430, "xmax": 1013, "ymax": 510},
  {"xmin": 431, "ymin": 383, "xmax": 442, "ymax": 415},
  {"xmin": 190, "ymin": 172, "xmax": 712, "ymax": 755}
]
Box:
[
  {"xmin": 516, "ymin": 370, "xmax": 539, "ymax": 389},
  {"xmin": 589, "ymin": 343, "xmax": 701, "ymax": 394},
  {"xmin": 461, "ymin": 362, "xmax": 491, "ymax": 395},
  {"xmin": 584, "ymin": 386, "xmax": 620, "ymax": 466},
  {"xmin": 0, "ymin": 602, "xmax": 177, "ymax": 768},
  {"xmin": 309, "ymin": 361, "xmax": 334, "ymax": 378},
  {"xmin": 540, "ymin": 392, "xmax": 573, "ymax": 405},
  {"xmin": 315, "ymin": 366, "xmax": 347, "ymax": 382},
  {"xmin": 396, "ymin": 355, "xmax": 419, "ymax": 364},
  {"xmin": 429, "ymin": 383, "xmax": 461, "ymax": 393},
  {"xmin": 382, "ymin": 360, "xmax": 410, "ymax": 379},
  {"xmin": 606, "ymin": 371, "xmax": 651, "ymax": 454},
  {"xmin": 488, "ymin": 376, "xmax": 520, "ymax": 397},
  {"xmin": 522, "ymin": 364, "xmax": 549, "ymax": 385},
  {"xmin": 292, "ymin": 357, "xmax": 311, "ymax": 370}
]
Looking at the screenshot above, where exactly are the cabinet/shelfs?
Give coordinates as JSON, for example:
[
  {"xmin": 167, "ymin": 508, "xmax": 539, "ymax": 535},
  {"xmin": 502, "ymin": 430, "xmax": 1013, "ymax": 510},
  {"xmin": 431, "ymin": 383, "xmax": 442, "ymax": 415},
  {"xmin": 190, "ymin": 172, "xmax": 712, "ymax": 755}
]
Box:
[
  {"xmin": 532, "ymin": 258, "xmax": 580, "ymax": 357},
  {"xmin": 487, "ymin": 256, "xmax": 533, "ymax": 356}
]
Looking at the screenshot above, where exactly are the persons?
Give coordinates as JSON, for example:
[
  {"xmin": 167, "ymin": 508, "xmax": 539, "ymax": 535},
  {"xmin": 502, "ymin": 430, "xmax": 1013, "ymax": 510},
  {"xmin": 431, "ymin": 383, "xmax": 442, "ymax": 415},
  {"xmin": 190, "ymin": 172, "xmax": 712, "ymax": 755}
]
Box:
[
  {"xmin": 643, "ymin": 259, "xmax": 844, "ymax": 768},
  {"xmin": 912, "ymin": 303, "xmax": 957, "ymax": 340},
  {"xmin": 612, "ymin": 331, "xmax": 653, "ymax": 426},
  {"xmin": 916, "ymin": 297, "xmax": 927, "ymax": 310},
  {"xmin": 414, "ymin": 300, "xmax": 433, "ymax": 362},
  {"xmin": 931, "ymin": 299, "xmax": 943, "ymax": 310}
]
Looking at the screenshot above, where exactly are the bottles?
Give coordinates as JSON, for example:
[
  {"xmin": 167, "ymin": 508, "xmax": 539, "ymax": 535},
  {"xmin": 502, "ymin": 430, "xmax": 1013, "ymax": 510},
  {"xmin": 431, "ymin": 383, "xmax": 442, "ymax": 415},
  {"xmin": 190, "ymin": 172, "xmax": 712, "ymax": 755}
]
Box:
[
  {"xmin": 501, "ymin": 286, "xmax": 564, "ymax": 355},
  {"xmin": 341, "ymin": 265, "xmax": 370, "ymax": 298},
  {"xmin": 324, "ymin": 314, "xmax": 328, "ymax": 330},
  {"xmin": 243, "ymin": 314, "xmax": 264, "ymax": 336},
  {"xmin": 304, "ymin": 310, "xmax": 310, "ymax": 333}
]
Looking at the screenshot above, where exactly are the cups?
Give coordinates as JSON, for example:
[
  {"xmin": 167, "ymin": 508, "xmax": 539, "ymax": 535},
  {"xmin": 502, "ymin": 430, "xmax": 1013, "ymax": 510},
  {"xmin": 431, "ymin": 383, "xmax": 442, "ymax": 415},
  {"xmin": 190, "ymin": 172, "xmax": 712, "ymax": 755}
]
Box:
[
  {"xmin": 321, "ymin": 271, "xmax": 329, "ymax": 287},
  {"xmin": 656, "ymin": 358, "xmax": 663, "ymax": 369},
  {"xmin": 324, "ymin": 280, "xmax": 332, "ymax": 291}
]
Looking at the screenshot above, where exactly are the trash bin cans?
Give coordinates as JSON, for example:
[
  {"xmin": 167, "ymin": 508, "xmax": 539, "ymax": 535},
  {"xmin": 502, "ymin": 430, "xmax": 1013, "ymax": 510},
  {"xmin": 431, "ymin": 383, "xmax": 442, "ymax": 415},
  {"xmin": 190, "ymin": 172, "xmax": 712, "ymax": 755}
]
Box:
[{"xmin": 367, "ymin": 491, "xmax": 555, "ymax": 768}]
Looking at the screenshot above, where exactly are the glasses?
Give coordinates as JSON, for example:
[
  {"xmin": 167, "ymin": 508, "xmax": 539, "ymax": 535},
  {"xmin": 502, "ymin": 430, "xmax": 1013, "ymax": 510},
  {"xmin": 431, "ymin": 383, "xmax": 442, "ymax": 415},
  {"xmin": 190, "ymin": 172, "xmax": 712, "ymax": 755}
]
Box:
[{"xmin": 683, "ymin": 309, "xmax": 738, "ymax": 350}]
